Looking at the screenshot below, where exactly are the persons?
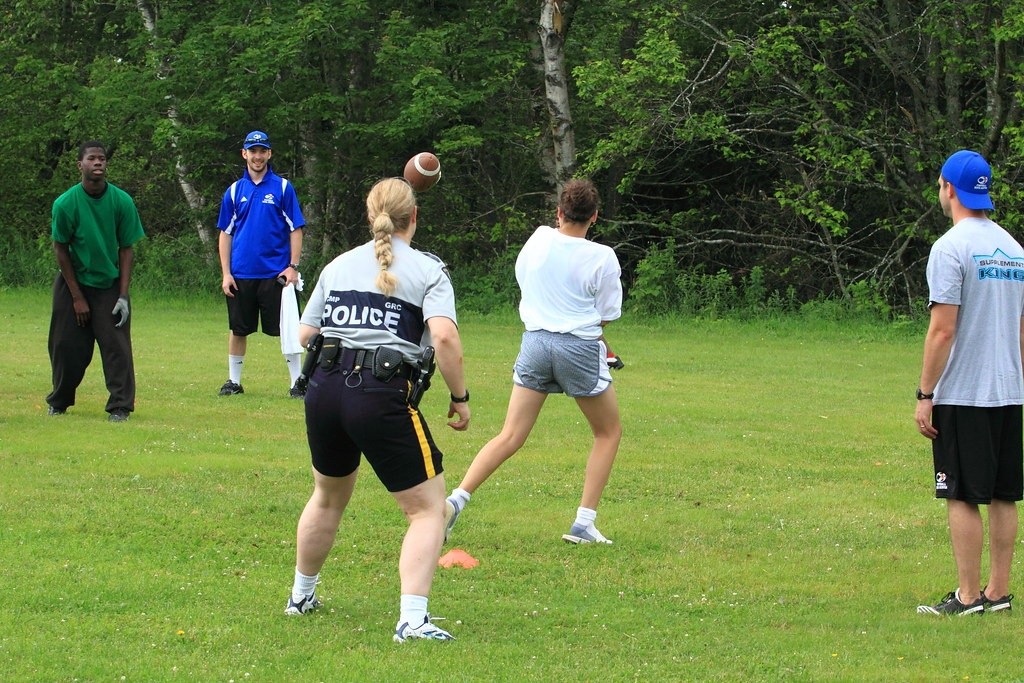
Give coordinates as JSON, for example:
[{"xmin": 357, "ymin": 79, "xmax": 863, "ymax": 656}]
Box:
[
  {"xmin": 445, "ymin": 182, "xmax": 622, "ymax": 544},
  {"xmin": 914, "ymin": 151, "xmax": 1024, "ymax": 616},
  {"xmin": 46, "ymin": 142, "xmax": 145, "ymax": 422},
  {"xmin": 285, "ymin": 179, "xmax": 471, "ymax": 642},
  {"xmin": 216, "ymin": 131, "xmax": 305, "ymax": 398}
]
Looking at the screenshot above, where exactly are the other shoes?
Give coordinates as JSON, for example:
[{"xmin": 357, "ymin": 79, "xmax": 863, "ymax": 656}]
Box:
[
  {"xmin": 47, "ymin": 405, "xmax": 66, "ymax": 417},
  {"xmin": 109, "ymin": 412, "xmax": 129, "ymax": 424}
]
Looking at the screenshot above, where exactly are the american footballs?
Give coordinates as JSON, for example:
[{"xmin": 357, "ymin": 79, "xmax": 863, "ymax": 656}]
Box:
[{"xmin": 404, "ymin": 152, "xmax": 441, "ymax": 193}]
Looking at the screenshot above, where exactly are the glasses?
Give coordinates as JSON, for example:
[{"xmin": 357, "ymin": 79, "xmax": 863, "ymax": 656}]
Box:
[
  {"xmin": 244, "ymin": 138, "xmax": 270, "ymax": 144},
  {"xmin": 372, "ymin": 177, "xmax": 411, "ymax": 188}
]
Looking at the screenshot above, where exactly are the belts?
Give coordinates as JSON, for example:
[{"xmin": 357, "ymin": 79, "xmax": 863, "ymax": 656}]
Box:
[{"xmin": 315, "ymin": 345, "xmax": 421, "ymax": 385}]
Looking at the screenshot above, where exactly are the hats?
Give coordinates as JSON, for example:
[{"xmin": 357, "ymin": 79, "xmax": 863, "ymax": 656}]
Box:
[
  {"xmin": 242, "ymin": 131, "xmax": 271, "ymax": 150},
  {"xmin": 941, "ymin": 150, "xmax": 995, "ymax": 212}
]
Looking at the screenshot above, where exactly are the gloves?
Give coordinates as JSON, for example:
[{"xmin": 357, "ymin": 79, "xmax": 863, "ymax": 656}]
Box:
[{"xmin": 112, "ymin": 295, "xmax": 129, "ymax": 328}]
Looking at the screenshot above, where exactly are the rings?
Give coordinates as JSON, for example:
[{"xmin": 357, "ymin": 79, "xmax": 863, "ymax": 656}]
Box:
[{"xmin": 921, "ymin": 425, "xmax": 925, "ymax": 428}]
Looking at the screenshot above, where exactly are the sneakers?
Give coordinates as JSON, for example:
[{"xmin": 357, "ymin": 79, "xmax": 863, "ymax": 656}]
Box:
[
  {"xmin": 979, "ymin": 585, "xmax": 1015, "ymax": 614},
  {"xmin": 561, "ymin": 519, "xmax": 613, "ymax": 545},
  {"xmin": 289, "ymin": 384, "xmax": 307, "ymax": 397},
  {"xmin": 284, "ymin": 588, "xmax": 324, "ymax": 616},
  {"xmin": 218, "ymin": 379, "xmax": 244, "ymax": 395},
  {"xmin": 931, "ymin": 587, "xmax": 985, "ymax": 617},
  {"xmin": 392, "ymin": 611, "xmax": 457, "ymax": 643},
  {"xmin": 444, "ymin": 493, "xmax": 464, "ymax": 545}
]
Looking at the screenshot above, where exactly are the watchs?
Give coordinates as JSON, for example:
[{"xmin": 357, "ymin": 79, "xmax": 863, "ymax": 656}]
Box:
[
  {"xmin": 289, "ymin": 263, "xmax": 299, "ymax": 271},
  {"xmin": 451, "ymin": 390, "xmax": 469, "ymax": 403},
  {"xmin": 916, "ymin": 389, "xmax": 934, "ymax": 400}
]
